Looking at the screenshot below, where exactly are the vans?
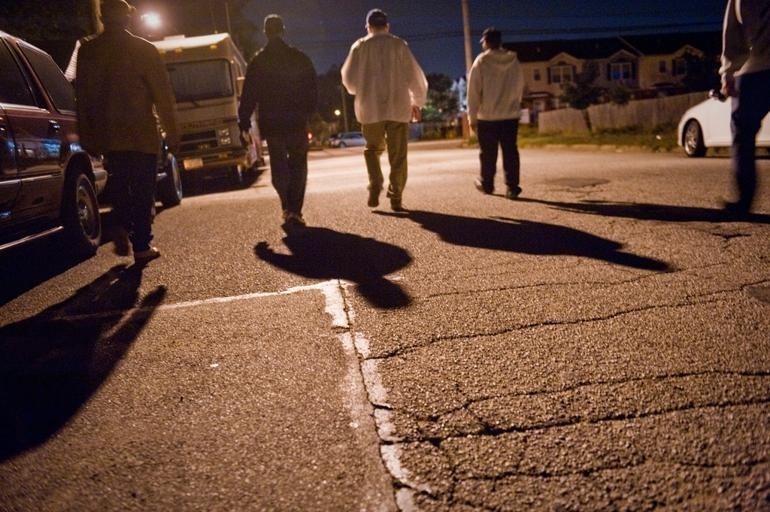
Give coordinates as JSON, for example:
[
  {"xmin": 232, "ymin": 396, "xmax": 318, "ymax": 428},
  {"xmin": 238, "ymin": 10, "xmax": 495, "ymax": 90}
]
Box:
[{"xmin": 1, "ymin": 30, "xmax": 107, "ymax": 264}]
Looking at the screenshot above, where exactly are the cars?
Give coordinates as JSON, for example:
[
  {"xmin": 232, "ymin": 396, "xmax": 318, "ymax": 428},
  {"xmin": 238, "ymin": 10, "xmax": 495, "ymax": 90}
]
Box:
[
  {"xmin": 327, "ymin": 131, "xmax": 367, "ymax": 148},
  {"xmin": 96, "ymin": 137, "xmax": 185, "ymax": 207},
  {"xmin": 676, "ymin": 85, "xmax": 770, "ymax": 157}
]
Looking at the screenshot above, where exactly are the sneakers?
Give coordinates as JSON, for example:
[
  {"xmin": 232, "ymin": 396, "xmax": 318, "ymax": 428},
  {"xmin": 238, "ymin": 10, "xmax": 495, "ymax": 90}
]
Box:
[
  {"xmin": 390, "ymin": 197, "xmax": 402, "ymax": 209},
  {"xmin": 474, "ymin": 177, "xmax": 485, "ymax": 191},
  {"xmin": 506, "ymin": 189, "xmax": 516, "ymax": 197},
  {"xmin": 112, "ymin": 225, "xmax": 128, "ymax": 256},
  {"xmin": 368, "ymin": 189, "xmax": 380, "ymax": 207},
  {"xmin": 134, "ymin": 243, "xmax": 160, "ymax": 266},
  {"xmin": 282, "ymin": 209, "xmax": 305, "ymax": 228}
]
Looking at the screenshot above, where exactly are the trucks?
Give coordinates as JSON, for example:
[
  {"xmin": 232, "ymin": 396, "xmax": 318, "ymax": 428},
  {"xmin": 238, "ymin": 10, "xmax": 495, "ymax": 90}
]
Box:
[{"xmin": 151, "ymin": 26, "xmax": 263, "ymax": 187}]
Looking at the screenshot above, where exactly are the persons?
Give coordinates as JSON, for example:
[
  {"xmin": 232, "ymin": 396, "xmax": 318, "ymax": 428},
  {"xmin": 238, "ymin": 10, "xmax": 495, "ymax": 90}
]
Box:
[
  {"xmin": 236, "ymin": 13, "xmax": 321, "ymax": 228},
  {"xmin": 464, "ymin": 26, "xmax": 525, "ymax": 199},
  {"xmin": 338, "ymin": 7, "xmax": 430, "ymax": 212},
  {"xmin": 74, "ymin": 2, "xmax": 185, "ymax": 265},
  {"xmin": 716, "ymin": 1, "xmax": 769, "ymax": 213}
]
